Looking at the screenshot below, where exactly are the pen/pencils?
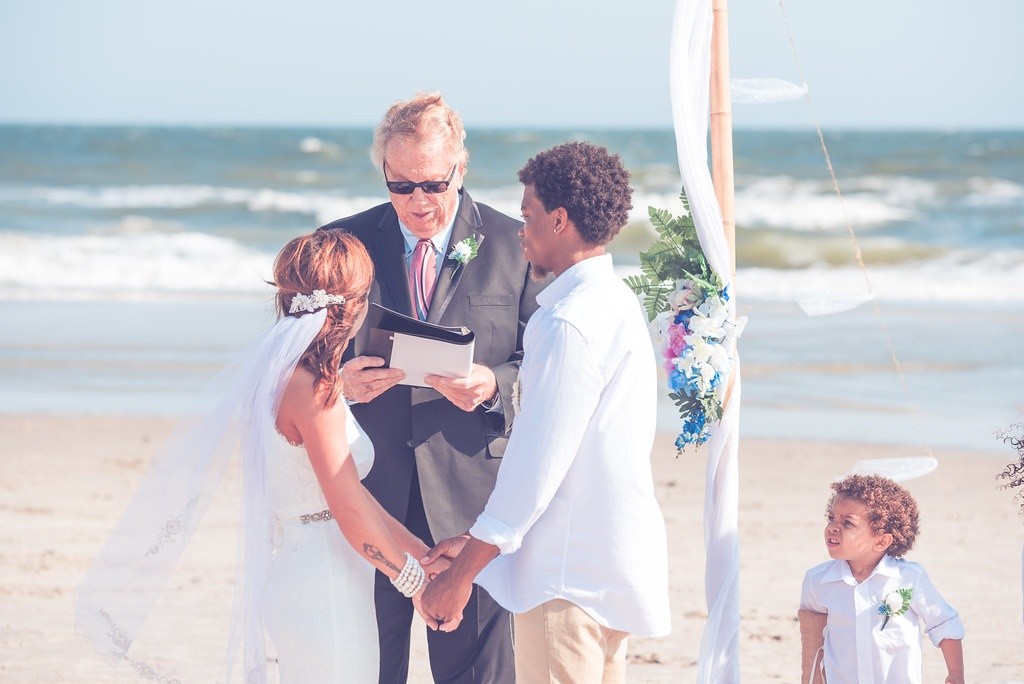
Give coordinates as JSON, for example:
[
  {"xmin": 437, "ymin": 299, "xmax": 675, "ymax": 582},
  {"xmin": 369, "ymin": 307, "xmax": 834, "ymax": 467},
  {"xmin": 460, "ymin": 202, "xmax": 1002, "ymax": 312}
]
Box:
[{"xmin": 879, "ymin": 612, "xmax": 891, "ymax": 633}]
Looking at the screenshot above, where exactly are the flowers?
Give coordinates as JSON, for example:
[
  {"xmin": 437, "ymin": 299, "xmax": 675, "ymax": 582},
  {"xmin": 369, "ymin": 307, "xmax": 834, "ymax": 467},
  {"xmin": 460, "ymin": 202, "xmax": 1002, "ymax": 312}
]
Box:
[
  {"xmin": 877, "ymin": 586, "xmax": 912, "ymax": 631},
  {"xmin": 447, "ymin": 233, "xmax": 480, "ymax": 279},
  {"xmin": 635, "ymin": 187, "xmax": 739, "ymax": 458}
]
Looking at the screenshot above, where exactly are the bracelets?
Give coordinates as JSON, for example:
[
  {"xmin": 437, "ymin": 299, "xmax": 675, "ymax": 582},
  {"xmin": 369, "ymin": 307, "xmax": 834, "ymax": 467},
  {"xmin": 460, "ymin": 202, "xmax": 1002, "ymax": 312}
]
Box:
[{"xmin": 390, "ymin": 553, "xmax": 424, "ymax": 598}]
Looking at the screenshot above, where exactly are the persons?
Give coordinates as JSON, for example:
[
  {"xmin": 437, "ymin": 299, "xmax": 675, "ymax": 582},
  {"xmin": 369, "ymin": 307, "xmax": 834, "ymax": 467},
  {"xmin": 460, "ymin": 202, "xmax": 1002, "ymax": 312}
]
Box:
[
  {"xmin": 799, "ymin": 474, "xmax": 965, "ymax": 684},
  {"xmin": 419, "ymin": 145, "xmax": 671, "ymax": 684},
  {"xmin": 75, "ymin": 229, "xmax": 463, "ymax": 684},
  {"xmin": 316, "ymin": 97, "xmax": 554, "ymax": 684}
]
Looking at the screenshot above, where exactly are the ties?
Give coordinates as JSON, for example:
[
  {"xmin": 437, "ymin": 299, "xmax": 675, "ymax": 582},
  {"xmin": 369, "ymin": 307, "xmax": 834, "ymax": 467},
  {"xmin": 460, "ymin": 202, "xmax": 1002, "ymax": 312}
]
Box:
[{"xmin": 409, "ymin": 238, "xmax": 437, "ymax": 322}]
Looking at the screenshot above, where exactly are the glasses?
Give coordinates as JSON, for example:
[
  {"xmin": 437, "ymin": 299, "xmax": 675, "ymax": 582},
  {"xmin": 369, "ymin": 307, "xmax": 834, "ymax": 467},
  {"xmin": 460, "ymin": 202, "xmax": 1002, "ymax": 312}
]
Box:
[{"xmin": 383, "ymin": 158, "xmax": 456, "ymax": 194}]
